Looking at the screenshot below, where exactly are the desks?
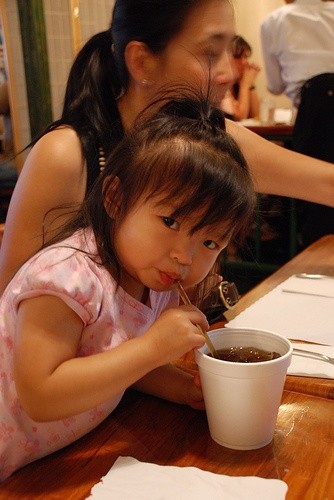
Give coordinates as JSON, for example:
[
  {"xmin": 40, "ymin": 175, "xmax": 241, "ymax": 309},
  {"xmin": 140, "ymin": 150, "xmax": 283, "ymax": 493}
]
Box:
[
  {"xmin": 0, "ymin": 233, "xmax": 334, "ymax": 500},
  {"xmin": 223, "ymin": 114, "xmax": 295, "ymax": 269}
]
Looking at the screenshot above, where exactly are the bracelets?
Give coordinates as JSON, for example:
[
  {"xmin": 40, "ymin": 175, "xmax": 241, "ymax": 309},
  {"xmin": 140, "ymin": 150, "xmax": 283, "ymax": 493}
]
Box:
[{"xmin": 250, "ymin": 86, "xmax": 255, "ymax": 90}]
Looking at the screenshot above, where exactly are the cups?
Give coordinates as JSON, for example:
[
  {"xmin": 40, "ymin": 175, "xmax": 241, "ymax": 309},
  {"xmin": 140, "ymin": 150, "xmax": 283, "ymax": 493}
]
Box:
[{"xmin": 194, "ymin": 328, "xmax": 293, "ymax": 450}]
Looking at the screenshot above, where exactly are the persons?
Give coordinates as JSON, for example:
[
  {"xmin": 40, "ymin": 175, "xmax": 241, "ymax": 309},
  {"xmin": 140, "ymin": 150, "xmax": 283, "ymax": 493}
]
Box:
[
  {"xmin": 218, "ymin": 34, "xmax": 260, "ymax": 121},
  {"xmin": 0, "ymin": 98, "xmax": 256, "ymax": 483},
  {"xmin": 0, "ymin": 0, "xmax": 334, "ymax": 297},
  {"xmin": 260, "ymin": 0, "xmax": 334, "ymax": 249}
]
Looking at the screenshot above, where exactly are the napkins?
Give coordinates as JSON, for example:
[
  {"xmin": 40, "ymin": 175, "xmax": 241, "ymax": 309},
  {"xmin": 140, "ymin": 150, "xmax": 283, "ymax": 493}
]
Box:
[{"xmin": 85, "ymin": 455, "xmax": 288, "ymax": 500}]
